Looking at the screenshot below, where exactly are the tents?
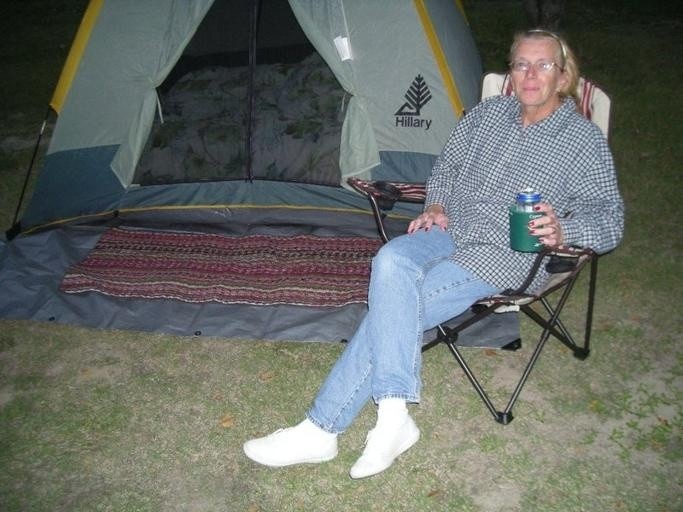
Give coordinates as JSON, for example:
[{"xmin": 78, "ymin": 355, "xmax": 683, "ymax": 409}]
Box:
[{"xmin": 4, "ymin": 1, "xmax": 482, "ymax": 240}]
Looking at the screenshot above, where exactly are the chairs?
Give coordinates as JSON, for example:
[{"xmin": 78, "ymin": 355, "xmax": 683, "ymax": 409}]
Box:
[{"xmin": 346, "ymin": 61, "xmax": 627, "ymax": 429}]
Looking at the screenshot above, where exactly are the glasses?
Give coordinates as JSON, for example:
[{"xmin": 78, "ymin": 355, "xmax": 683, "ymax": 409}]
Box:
[{"xmin": 508, "ymin": 59, "xmax": 564, "ymax": 73}]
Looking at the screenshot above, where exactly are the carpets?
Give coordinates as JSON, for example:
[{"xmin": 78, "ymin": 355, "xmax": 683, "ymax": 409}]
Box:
[{"xmin": 59, "ymin": 225, "xmax": 391, "ymax": 308}]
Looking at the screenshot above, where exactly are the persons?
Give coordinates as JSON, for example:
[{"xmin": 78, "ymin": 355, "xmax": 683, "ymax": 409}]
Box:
[{"xmin": 242, "ymin": 30, "xmax": 626, "ymax": 477}]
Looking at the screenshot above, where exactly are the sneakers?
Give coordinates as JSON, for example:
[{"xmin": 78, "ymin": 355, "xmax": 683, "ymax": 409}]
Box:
[
  {"xmin": 349, "ymin": 413, "xmax": 421, "ymax": 480},
  {"xmin": 243, "ymin": 418, "xmax": 339, "ymax": 467}
]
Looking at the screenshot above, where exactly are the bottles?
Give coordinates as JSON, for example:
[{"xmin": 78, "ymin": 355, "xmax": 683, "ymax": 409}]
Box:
[{"xmin": 509, "ymin": 192, "xmax": 547, "ymax": 253}]
